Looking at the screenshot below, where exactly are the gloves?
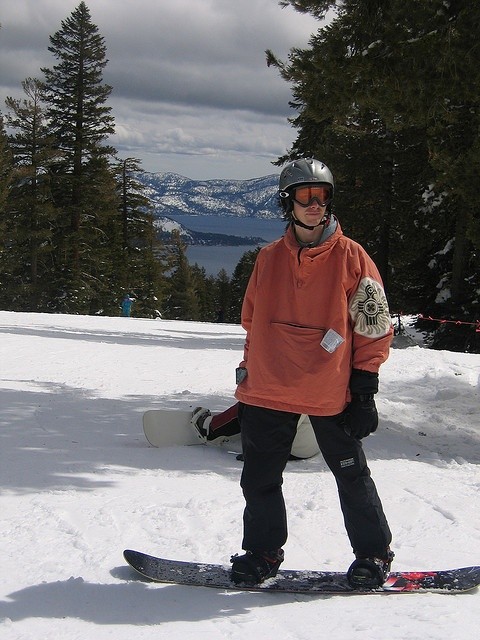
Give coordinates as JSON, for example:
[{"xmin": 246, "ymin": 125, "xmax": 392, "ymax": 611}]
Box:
[
  {"xmin": 237, "ymin": 400, "xmax": 244, "ymax": 426},
  {"xmin": 348, "ymin": 399, "xmax": 378, "ymax": 441}
]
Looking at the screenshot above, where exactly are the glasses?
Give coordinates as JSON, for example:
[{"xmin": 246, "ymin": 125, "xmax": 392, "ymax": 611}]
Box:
[{"xmin": 289, "ymin": 184, "xmax": 335, "ymax": 207}]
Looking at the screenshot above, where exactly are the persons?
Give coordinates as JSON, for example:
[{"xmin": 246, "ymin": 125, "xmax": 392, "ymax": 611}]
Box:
[{"xmin": 231, "ymin": 158, "xmax": 395, "ymax": 589}]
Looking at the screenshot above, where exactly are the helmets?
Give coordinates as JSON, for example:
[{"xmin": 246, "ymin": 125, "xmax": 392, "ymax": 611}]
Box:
[{"xmin": 279, "ymin": 158, "xmax": 334, "ymax": 216}]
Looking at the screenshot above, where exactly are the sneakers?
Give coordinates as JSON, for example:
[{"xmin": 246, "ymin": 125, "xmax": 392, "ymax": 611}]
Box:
[
  {"xmin": 193, "ymin": 407, "xmax": 220, "ymax": 441},
  {"xmin": 351, "ymin": 545, "xmax": 395, "ymax": 586},
  {"xmin": 232, "ymin": 549, "xmax": 284, "ymax": 580}
]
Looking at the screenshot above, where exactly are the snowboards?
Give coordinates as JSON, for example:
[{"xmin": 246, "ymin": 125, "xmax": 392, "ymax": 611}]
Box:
[
  {"xmin": 141, "ymin": 410, "xmax": 223, "ymax": 449},
  {"xmin": 122, "ymin": 549, "xmax": 480, "ymax": 594}
]
